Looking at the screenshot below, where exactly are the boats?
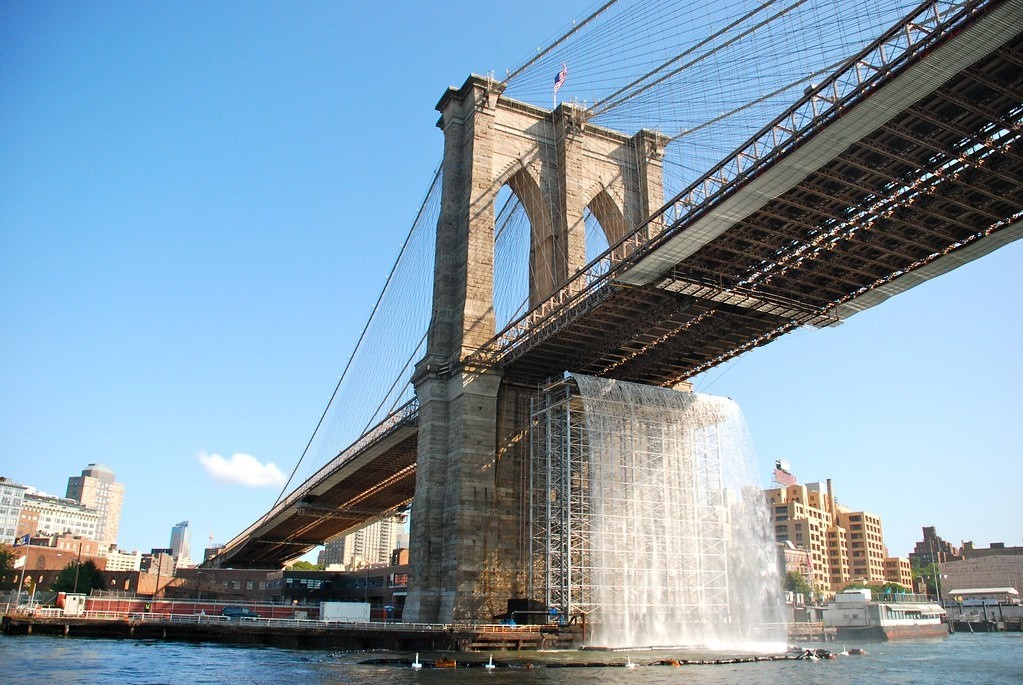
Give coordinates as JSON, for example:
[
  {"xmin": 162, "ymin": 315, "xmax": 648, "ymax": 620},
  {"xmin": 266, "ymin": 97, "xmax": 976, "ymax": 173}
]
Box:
[{"xmin": 823, "ymin": 589, "xmax": 949, "ymax": 642}]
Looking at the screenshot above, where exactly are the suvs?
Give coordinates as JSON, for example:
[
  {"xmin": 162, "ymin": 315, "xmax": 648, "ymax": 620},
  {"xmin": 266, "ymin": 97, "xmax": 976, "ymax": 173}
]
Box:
[{"xmin": 221, "ymin": 607, "xmax": 262, "ymax": 622}]
